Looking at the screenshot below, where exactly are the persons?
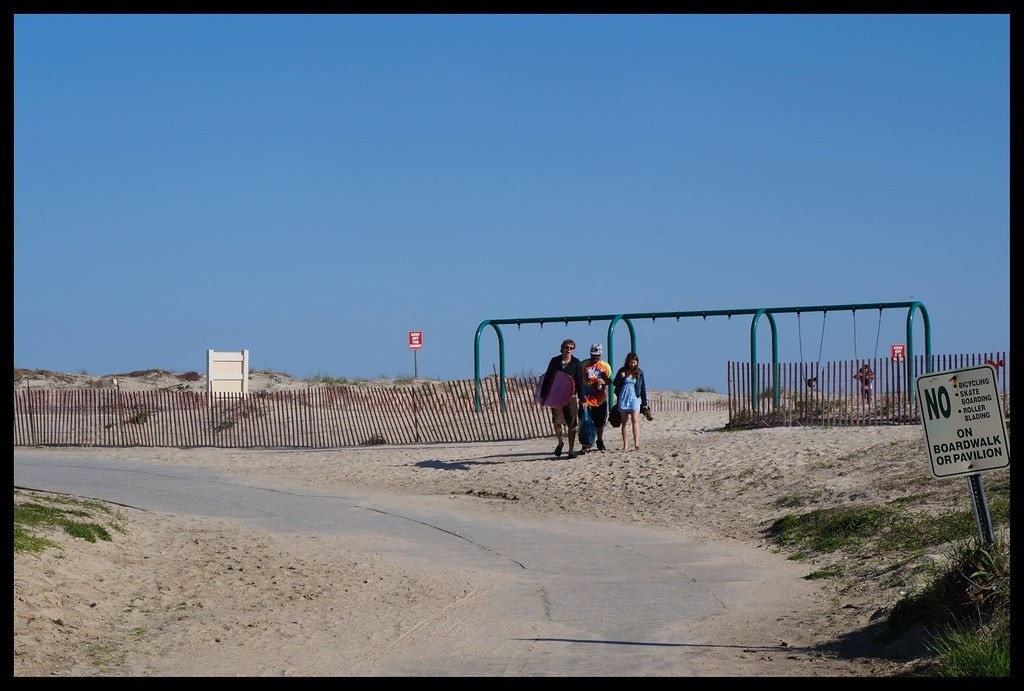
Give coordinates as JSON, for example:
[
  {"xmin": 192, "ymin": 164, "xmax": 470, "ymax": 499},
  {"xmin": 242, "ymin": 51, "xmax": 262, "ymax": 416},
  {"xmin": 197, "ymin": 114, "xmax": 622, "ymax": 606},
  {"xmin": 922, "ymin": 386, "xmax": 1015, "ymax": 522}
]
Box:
[
  {"xmin": 855, "ymin": 365, "xmax": 875, "ymax": 404},
  {"xmin": 805, "ymin": 379, "xmax": 816, "ymax": 388},
  {"xmin": 613, "ymin": 352, "xmax": 648, "ymax": 452},
  {"xmin": 580, "ymin": 343, "xmax": 614, "ymax": 455},
  {"xmin": 540, "ymin": 338, "xmax": 587, "ymax": 460}
]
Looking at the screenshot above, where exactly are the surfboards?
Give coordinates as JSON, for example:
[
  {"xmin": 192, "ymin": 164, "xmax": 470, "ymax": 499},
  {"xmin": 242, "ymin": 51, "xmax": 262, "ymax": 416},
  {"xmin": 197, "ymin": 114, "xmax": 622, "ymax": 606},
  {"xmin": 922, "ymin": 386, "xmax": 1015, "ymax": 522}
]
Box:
[{"xmin": 534, "ymin": 371, "xmax": 576, "ymax": 409}]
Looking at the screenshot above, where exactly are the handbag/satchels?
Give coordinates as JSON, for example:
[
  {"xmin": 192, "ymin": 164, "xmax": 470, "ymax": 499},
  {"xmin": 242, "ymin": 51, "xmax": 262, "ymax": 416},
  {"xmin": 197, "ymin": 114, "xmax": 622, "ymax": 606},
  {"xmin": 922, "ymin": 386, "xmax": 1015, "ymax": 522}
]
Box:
[
  {"xmin": 578, "ymin": 407, "xmax": 595, "ymax": 446},
  {"xmin": 608, "ymin": 404, "xmax": 623, "ymax": 428}
]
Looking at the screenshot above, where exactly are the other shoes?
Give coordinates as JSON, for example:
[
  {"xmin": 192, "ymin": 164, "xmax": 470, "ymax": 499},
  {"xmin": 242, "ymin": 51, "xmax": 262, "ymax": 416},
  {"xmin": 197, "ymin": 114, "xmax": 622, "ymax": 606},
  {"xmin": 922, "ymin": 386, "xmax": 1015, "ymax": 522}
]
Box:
[
  {"xmin": 596, "ymin": 439, "xmax": 605, "ymax": 450},
  {"xmin": 643, "ymin": 407, "xmax": 654, "ymax": 422},
  {"xmin": 566, "ymin": 452, "xmax": 577, "ymax": 459},
  {"xmin": 579, "ymin": 446, "xmax": 589, "ymax": 456},
  {"xmin": 554, "ymin": 442, "xmax": 564, "ymax": 456}
]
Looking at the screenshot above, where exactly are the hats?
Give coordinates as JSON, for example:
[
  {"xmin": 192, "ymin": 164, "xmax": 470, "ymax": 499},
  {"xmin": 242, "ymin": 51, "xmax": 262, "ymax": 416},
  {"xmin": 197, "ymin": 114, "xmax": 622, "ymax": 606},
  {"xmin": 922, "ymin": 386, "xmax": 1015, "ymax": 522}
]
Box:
[{"xmin": 590, "ymin": 343, "xmax": 602, "ymax": 355}]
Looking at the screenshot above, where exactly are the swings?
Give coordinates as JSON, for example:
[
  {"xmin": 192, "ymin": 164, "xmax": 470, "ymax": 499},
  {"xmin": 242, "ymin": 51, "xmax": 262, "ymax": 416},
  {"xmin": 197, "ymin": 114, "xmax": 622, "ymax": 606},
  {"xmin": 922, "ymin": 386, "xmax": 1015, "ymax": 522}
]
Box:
[
  {"xmin": 796, "ymin": 305, "xmax": 827, "ymax": 387},
  {"xmin": 851, "ymin": 302, "xmax": 883, "ymax": 385}
]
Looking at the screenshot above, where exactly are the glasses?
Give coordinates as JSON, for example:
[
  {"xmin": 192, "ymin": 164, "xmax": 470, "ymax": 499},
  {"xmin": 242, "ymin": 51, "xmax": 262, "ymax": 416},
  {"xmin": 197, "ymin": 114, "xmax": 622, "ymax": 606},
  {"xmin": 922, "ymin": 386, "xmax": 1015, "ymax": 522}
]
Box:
[{"xmin": 564, "ymin": 346, "xmax": 574, "ymax": 350}]
